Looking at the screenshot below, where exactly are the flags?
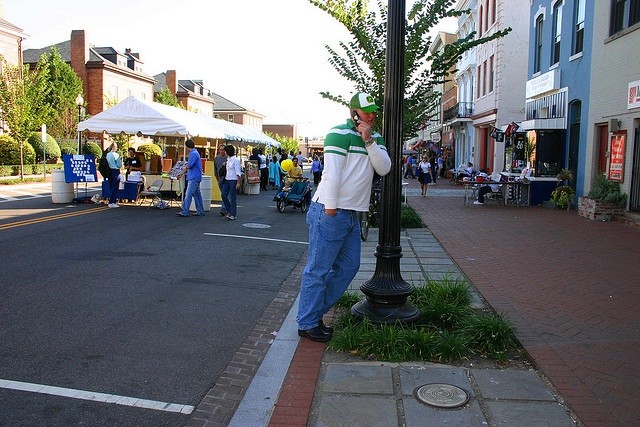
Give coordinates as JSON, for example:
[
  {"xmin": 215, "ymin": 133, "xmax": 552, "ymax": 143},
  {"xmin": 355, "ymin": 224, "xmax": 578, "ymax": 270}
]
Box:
[
  {"xmin": 489, "ymin": 128, "xmax": 501, "ymax": 139},
  {"xmin": 496, "ymin": 131, "xmax": 504, "ymax": 142},
  {"xmin": 505, "ymin": 122, "xmax": 519, "ymax": 137}
]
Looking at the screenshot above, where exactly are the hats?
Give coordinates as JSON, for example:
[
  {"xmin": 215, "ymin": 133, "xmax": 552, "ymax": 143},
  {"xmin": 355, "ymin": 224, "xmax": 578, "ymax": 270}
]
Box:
[{"xmin": 350, "ymin": 92, "xmax": 380, "ymax": 113}]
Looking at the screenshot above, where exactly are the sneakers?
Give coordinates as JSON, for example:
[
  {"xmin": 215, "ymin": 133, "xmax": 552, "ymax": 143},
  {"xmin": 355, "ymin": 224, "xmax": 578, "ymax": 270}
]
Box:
[
  {"xmin": 108, "ymin": 203, "xmax": 119, "ymax": 208},
  {"xmin": 229, "ymin": 216, "xmax": 238, "ymax": 219},
  {"xmin": 193, "ymin": 212, "xmax": 205, "ymax": 216},
  {"xmin": 176, "ymin": 212, "xmax": 189, "ymax": 216},
  {"xmin": 220, "ymin": 208, "xmax": 225, "ymax": 216},
  {"xmin": 226, "ymin": 213, "xmax": 231, "ymax": 218},
  {"xmin": 473, "ymin": 200, "xmax": 483, "ymax": 205}
]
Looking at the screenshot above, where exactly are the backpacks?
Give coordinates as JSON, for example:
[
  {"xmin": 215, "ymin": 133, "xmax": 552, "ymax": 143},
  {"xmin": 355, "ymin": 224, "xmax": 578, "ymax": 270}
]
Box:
[{"xmin": 99, "ymin": 152, "xmax": 112, "ymax": 178}]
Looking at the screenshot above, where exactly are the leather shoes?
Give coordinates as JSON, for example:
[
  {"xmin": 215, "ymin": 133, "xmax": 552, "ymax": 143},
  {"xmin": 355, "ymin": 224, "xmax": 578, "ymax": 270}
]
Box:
[
  {"xmin": 319, "ymin": 320, "xmax": 333, "ymax": 333},
  {"xmin": 298, "ymin": 326, "xmax": 330, "ymax": 342}
]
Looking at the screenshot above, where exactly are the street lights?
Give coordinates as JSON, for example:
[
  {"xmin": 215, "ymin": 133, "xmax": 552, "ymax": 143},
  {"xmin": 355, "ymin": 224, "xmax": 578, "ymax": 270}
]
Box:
[{"xmin": 76, "ymin": 94, "xmax": 84, "ymax": 154}]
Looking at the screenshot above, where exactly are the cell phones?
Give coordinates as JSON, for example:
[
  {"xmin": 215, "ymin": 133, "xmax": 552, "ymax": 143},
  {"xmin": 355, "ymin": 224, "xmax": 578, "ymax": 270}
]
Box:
[{"xmin": 351, "ymin": 110, "xmax": 362, "ymax": 128}]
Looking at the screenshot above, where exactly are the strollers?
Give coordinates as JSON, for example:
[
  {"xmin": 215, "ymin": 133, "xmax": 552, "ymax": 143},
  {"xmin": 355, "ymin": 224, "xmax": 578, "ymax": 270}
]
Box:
[{"xmin": 274, "ymin": 176, "xmax": 310, "ymax": 212}]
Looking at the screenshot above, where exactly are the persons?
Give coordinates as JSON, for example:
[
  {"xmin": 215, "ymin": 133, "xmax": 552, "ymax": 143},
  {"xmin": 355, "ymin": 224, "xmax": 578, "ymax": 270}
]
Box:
[
  {"xmin": 221, "ymin": 145, "xmax": 241, "ymax": 220},
  {"xmin": 281, "ymin": 149, "xmax": 287, "ymax": 159},
  {"xmin": 429, "ymin": 154, "xmax": 438, "ymax": 186},
  {"xmin": 288, "ymin": 157, "xmax": 302, "ymax": 210},
  {"xmin": 474, "ymin": 169, "xmax": 498, "ymax": 205},
  {"xmin": 295, "ymin": 92, "xmax": 392, "ymax": 343},
  {"xmin": 437, "ymin": 153, "xmax": 446, "ymax": 179},
  {"xmin": 404, "ymin": 153, "xmax": 417, "ymax": 179},
  {"xmin": 404, "ymin": 155, "xmax": 409, "ymax": 172},
  {"xmin": 214, "ymin": 144, "xmax": 231, "ymax": 215},
  {"xmin": 288, "ymin": 150, "xmax": 295, "ymax": 159},
  {"xmin": 310, "ymin": 157, "xmax": 322, "ymax": 186},
  {"xmin": 296, "ymin": 150, "xmax": 305, "ymax": 172},
  {"xmin": 106, "ymin": 142, "xmax": 121, "ymax": 209},
  {"xmin": 457, "ymin": 162, "xmax": 473, "ymax": 180},
  {"xmin": 123, "ymin": 147, "xmax": 143, "ymax": 202},
  {"xmin": 269, "ymin": 156, "xmax": 281, "ymax": 190},
  {"xmin": 250, "ymin": 148, "xmax": 261, "ymax": 170},
  {"xmin": 274, "ymin": 181, "xmax": 292, "ymax": 198},
  {"xmin": 313, "ymin": 152, "xmax": 321, "ymax": 181},
  {"xmin": 176, "ymin": 139, "xmax": 206, "ymax": 217},
  {"xmin": 280, "ymin": 153, "xmax": 292, "ymax": 174},
  {"xmin": 418, "ymin": 155, "xmax": 433, "ymax": 197},
  {"xmin": 412, "ymin": 156, "xmax": 417, "ymax": 176},
  {"xmin": 258, "ymin": 147, "xmax": 268, "ymax": 191},
  {"xmin": 276, "ymin": 147, "xmax": 283, "ymax": 160}
]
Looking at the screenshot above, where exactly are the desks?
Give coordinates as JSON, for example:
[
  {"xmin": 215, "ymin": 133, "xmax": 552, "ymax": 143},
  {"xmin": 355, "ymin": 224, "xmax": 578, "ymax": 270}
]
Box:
[
  {"xmin": 459, "ymin": 179, "xmax": 525, "ymax": 208},
  {"xmin": 401, "ymin": 183, "xmax": 410, "ymax": 202}
]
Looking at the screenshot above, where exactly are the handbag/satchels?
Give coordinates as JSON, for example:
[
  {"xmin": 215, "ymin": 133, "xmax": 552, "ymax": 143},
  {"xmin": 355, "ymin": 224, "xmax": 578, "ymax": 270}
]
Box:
[
  {"xmin": 219, "ymin": 166, "xmax": 225, "ymax": 177},
  {"xmin": 418, "ymin": 173, "xmax": 422, "ymax": 182}
]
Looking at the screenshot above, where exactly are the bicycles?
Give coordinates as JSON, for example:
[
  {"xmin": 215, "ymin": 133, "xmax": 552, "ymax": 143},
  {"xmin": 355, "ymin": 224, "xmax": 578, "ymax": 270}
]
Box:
[{"xmin": 359, "ymin": 188, "xmax": 382, "ymax": 240}]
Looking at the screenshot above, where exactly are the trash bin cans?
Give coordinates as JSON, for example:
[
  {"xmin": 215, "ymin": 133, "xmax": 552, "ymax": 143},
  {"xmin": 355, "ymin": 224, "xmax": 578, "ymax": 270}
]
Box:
[{"xmin": 122, "ymin": 181, "xmax": 138, "ymax": 202}]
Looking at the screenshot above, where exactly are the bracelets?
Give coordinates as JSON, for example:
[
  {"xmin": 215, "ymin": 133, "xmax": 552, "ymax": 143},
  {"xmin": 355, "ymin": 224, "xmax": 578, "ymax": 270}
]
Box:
[{"xmin": 365, "ymin": 139, "xmax": 373, "ymax": 146}]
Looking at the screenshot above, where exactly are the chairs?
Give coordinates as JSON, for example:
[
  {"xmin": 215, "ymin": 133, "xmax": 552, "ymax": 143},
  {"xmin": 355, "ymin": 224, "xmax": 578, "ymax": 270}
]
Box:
[
  {"xmin": 485, "ymin": 184, "xmax": 504, "ymax": 205},
  {"xmin": 470, "ymin": 184, "xmax": 479, "ymax": 201},
  {"xmin": 139, "ymin": 178, "xmax": 163, "ymax": 211},
  {"xmin": 455, "ymin": 169, "xmax": 477, "ymax": 184}
]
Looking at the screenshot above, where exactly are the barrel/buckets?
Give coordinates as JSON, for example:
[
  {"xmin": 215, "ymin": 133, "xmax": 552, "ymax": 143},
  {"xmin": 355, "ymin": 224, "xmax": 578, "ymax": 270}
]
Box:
[
  {"xmin": 189, "ymin": 177, "xmax": 212, "ymax": 212},
  {"xmin": 52, "ymin": 172, "xmax": 74, "ymax": 204},
  {"xmin": 244, "ymin": 169, "xmax": 260, "ymax": 195}
]
